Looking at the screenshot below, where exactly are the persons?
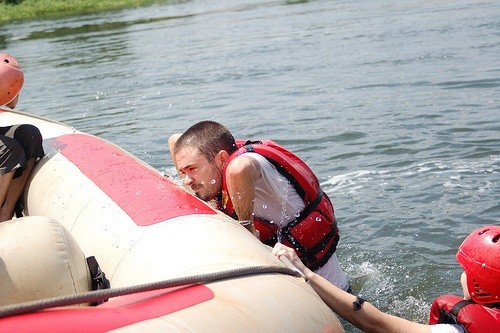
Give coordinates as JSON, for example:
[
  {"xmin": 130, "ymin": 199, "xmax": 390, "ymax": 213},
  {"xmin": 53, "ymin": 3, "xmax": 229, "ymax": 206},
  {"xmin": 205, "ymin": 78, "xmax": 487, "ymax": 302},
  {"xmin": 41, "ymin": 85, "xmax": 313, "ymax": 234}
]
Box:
[
  {"xmin": 0, "ymin": 53, "xmax": 45, "ymax": 223},
  {"xmin": 272, "ymin": 225, "xmax": 500, "ymax": 333},
  {"xmin": 168, "ymin": 120, "xmax": 352, "ymax": 306}
]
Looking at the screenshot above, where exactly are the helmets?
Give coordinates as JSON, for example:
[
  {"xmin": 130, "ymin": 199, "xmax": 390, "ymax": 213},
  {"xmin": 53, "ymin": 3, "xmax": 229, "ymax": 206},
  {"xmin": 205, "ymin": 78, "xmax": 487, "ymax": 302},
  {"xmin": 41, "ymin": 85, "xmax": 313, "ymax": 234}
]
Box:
[{"xmin": 456, "ymin": 225, "xmax": 500, "ymax": 303}]
